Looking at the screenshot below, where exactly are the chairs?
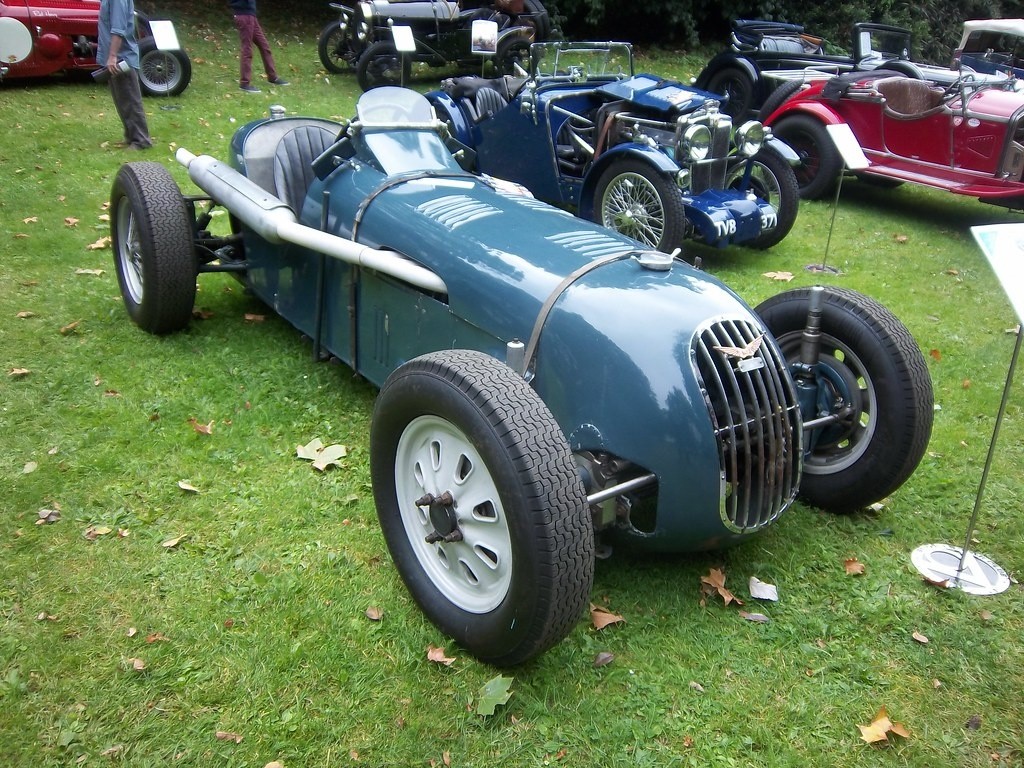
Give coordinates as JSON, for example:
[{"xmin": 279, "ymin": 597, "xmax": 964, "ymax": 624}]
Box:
[
  {"xmin": 274, "ymin": 124, "xmax": 337, "ymax": 208},
  {"xmin": 476, "ymin": 86, "xmax": 507, "ymax": 118}
]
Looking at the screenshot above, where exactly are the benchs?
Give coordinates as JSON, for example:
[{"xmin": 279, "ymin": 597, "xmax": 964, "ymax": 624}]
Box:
[{"xmin": 758, "ymin": 37, "xmax": 804, "ymax": 52}]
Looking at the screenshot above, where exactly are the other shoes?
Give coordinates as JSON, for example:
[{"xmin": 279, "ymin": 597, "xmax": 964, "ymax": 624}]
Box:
[
  {"xmin": 125, "ymin": 145, "xmax": 142, "ymax": 151},
  {"xmin": 114, "ymin": 141, "xmax": 130, "ymax": 147}
]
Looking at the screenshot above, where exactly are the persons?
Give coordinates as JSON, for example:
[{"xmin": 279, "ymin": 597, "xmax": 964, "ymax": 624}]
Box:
[
  {"xmin": 229, "ymin": 0, "xmax": 290, "ymax": 92},
  {"xmin": 96, "ymin": 0, "xmax": 153, "ymax": 150}
]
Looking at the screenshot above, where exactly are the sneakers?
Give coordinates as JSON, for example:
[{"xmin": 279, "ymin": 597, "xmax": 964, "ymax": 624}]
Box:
[
  {"xmin": 239, "ymin": 85, "xmax": 262, "ymax": 93},
  {"xmin": 267, "ymin": 77, "xmax": 290, "ymax": 85}
]
